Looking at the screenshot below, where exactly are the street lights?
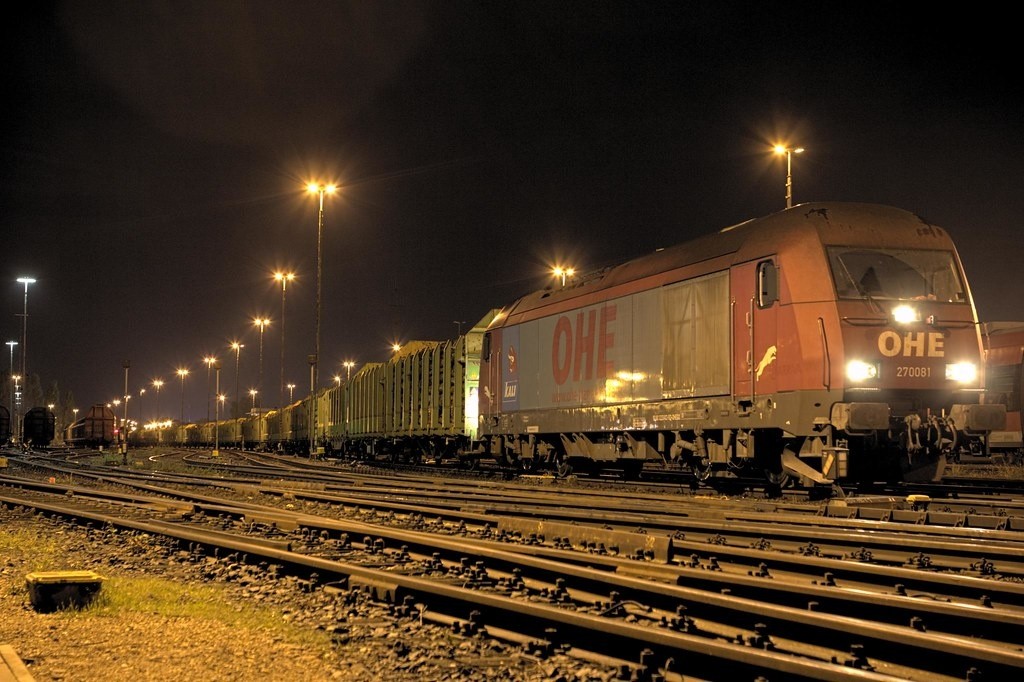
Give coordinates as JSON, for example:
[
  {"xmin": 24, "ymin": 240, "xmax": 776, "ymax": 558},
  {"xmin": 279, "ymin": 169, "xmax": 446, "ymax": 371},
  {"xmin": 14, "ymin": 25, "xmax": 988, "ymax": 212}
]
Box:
[
  {"xmin": 140, "ymin": 389, "xmax": 145, "ymax": 429},
  {"xmin": 178, "ymin": 369, "xmax": 188, "ymax": 448},
  {"xmin": 255, "ymin": 318, "xmax": 269, "ymax": 452},
  {"xmin": 233, "ymin": 342, "xmax": 244, "ymax": 450},
  {"xmin": 205, "ymin": 358, "xmax": 215, "ymax": 450},
  {"xmin": 775, "ymin": 145, "xmax": 805, "ymax": 208},
  {"xmin": 17, "ymin": 278, "xmax": 37, "ymax": 446},
  {"xmin": 6, "ymin": 341, "xmax": 21, "ymax": 440},
  {"xmin": 276, "ymin": 272, "xmax": 294, "ymax": 453},
  {"xmin": 154, "ymin": 380, "xmax": 163, "ymax": 447},
  {"xmin": 73, "ymin": 408, "xmax": 79, "ymax": 422},
  {"xmin": 306, "ymin": 179, "xmax": 338, "ymax": 460}
]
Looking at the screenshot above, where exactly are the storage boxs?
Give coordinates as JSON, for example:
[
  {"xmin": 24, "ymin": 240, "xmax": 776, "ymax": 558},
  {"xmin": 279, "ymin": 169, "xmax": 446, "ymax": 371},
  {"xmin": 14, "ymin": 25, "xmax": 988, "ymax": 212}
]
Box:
[
  {"xmin": 212, "ymin": 451, "xmax": 219, "ymax": 457},
  {"xmin": 24, "ymin": 571, "xmax": 107, "ymax": 614},
  {"xmin": 906, "ymin": 494, "xmax": 932, "ymax": 509},
  {"xmin": 316, "ymin": 447, "xmax": 325, "ymax": 454}
]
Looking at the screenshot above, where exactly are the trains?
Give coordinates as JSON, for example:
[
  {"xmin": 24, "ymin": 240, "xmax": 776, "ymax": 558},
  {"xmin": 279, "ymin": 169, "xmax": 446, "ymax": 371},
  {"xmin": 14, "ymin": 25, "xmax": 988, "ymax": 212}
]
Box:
[
  {"xmin": 945, "ymin": 322, "xmax": 1024, "ymax": 463},
  {"xmin": 132, "ymin": 203, "xmax": 988, "ymax": 489},
  {"xmin": 23, "ymin": 407, "xmax": 55, "ymax": 445},
  {"xmin": 64, "ymin": 404, "xmax": 114, "ymax": 448}
]
